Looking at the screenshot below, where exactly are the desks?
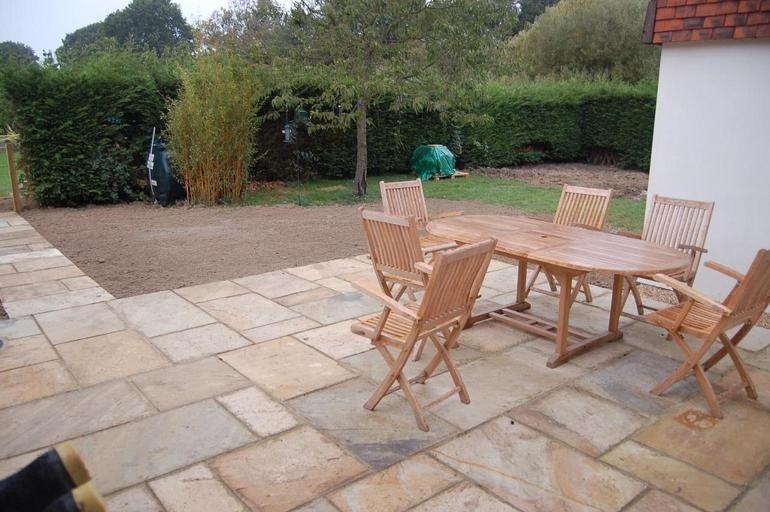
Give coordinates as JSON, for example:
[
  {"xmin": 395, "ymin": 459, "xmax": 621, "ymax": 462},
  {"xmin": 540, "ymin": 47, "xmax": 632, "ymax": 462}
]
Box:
[{"xmin": 424, "ymin": 214, "xmax": 692, "ymax": 369}]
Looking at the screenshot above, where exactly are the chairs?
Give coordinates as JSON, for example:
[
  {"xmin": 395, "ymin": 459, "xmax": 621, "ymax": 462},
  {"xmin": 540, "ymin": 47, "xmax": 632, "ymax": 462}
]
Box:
[{"xmin": 349, "ymin": 174, "xmax": 498, "ymax": 436}]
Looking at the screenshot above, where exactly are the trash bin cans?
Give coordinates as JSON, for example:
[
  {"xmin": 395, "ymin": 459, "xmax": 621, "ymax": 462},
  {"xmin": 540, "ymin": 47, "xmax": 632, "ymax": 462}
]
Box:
[{"xmin": 151, "ymin": 141, "xmax": 187, "ymax": 207}]
